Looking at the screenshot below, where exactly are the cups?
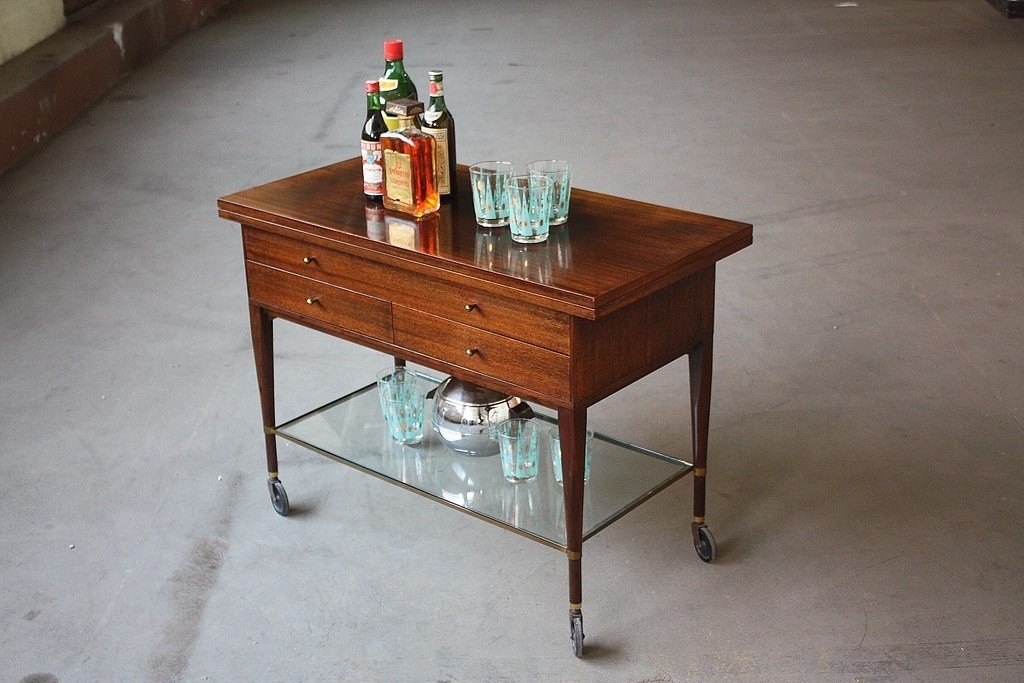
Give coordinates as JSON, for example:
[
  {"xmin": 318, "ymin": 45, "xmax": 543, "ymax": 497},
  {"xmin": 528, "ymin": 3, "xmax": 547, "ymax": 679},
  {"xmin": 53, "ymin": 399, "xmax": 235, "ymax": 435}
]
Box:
[
  {"xmin": 376, "ymin": 366, "xmax": 417, "ymax": 423},
  {"xmin": 548, "ymin": 425, "xmax": 594, "ymax": 488},
  {"xmin": 498, "ymin": 418, "xmax": 542, "ymax": 483},
  {"xmin": 469, "ymin": 161, "xmax": 515, "ymax": 227},
  {"xmin": 382, "ymin": 384, "xmax": 425, "ymax": 445},
  {"xmin": 527, "ymin": 159, "xmax": 572, "ymax": 225},
  {"xmin": 506, "ymin": 175, "xmax": 554, "ymax": 244}
]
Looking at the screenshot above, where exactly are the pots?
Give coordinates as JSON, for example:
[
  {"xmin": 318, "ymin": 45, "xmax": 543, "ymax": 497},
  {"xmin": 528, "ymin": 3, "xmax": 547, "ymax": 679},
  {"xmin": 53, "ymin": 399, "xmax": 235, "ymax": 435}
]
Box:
[{"xmin": 426, "ymin": 376, "xmax": 535, "ymax": 457}]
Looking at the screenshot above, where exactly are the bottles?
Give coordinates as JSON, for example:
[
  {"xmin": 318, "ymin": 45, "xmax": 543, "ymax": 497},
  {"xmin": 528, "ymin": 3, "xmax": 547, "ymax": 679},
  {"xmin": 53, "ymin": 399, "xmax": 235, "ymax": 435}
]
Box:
[
  {"xmin": 361, "ymin": 80, "xmax": 388, "ymax": 201},
  {"xmin": 423, "ymin": 70, "xmax": 457, "ymax": 204},
  {"xmin": 378, "ymin": 40, "xmax": 422, "ymax": 132},
  {"xmin": 380, "ymin": 98, "xmax": 441, "ymax": 217}
]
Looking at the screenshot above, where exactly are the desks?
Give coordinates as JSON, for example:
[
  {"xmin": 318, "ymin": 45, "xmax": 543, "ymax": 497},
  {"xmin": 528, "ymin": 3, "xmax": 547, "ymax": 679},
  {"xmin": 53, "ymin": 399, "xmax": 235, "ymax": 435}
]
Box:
[{"xmin": 216, "ymin": 154, "xmax": 751, "ymax": 625}]
